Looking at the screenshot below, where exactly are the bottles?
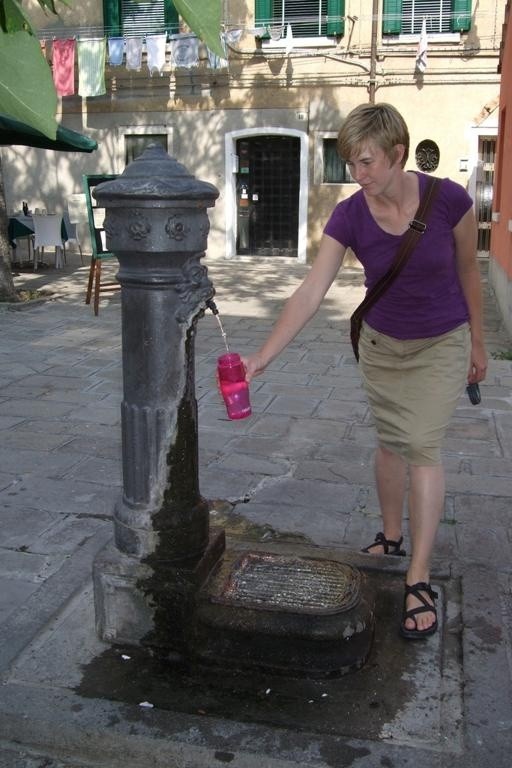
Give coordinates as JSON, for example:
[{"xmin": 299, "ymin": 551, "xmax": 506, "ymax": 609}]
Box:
[{"xmin": 23, "ymin": 202, "xmax": 29, "ymax": 217}]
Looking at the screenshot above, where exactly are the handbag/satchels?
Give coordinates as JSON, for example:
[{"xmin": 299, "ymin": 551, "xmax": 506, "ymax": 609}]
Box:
[{"xmin": 350, "ymin": 315, "xmax": 362, "ymax": 362}]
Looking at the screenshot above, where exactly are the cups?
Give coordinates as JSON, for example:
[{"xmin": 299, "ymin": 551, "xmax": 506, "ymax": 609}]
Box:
[
  {"xmin": 214, "ymin": 351, "xmax": 251, "ymax": 425},
  {"xmin": 41, "ymin": 209, "xmax": 46, "ymax": 216},
  {"xmin": 34, "ymin": 207, "xmax": 40, "ymax": 215}
]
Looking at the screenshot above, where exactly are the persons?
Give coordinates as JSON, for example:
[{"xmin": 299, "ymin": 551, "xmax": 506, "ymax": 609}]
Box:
[{"xmin": 213, "ymin": 100, "xmax": 490, "ymax": 642}]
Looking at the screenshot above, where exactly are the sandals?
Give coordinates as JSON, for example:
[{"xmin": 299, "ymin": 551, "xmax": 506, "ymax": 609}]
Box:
[
  {"xmin": 399, "ymin": 581, "xmax": 439, "ymax": 638},
  {"xmin": 361, "ymin": 531, "xmax": 407, "ymax": 556}
]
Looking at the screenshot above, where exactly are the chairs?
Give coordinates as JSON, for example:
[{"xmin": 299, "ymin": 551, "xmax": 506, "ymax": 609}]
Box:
[{"xmin": 7, "ymin": 208, "xmax": 84, "ymax": 273}]
